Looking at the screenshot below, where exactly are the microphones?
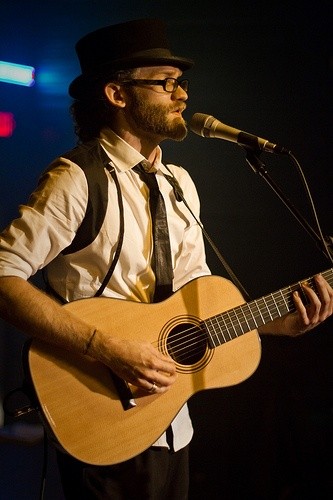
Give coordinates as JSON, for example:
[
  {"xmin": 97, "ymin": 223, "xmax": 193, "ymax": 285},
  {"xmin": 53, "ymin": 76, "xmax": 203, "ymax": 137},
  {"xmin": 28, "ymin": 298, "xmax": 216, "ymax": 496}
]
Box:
[{"xmin": 190, "ymin": 113, "xmax": 290, "ymax": 155}]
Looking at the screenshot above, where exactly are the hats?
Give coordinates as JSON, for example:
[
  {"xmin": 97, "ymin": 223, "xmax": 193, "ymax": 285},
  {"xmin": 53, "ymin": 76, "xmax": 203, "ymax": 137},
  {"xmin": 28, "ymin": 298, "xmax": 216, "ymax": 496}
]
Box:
[{"xmin": 69, "ymin": 19, "xmax": 194, "ymax": 98}]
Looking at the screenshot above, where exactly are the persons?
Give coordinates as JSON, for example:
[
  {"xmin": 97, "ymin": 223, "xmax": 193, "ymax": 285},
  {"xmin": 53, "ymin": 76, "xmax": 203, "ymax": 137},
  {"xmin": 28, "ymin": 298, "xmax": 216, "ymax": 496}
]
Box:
[{"xmin": 0, "ymin": 18, "xmax": 332, "ymax": 500}]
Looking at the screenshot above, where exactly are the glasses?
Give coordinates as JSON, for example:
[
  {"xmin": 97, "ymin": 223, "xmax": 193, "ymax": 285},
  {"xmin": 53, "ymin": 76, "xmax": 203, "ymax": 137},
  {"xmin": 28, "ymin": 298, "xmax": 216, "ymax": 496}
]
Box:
[{"xmin": 111, "ymin": 77, "xmax": 188, "ymax": 93}]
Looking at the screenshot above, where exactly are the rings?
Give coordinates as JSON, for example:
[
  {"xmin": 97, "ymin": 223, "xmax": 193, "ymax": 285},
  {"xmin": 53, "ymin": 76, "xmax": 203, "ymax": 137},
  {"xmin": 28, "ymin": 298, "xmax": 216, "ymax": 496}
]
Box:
[{"xmin": 146, "ymin": 383, "xmax": 158, "ymax": 394}]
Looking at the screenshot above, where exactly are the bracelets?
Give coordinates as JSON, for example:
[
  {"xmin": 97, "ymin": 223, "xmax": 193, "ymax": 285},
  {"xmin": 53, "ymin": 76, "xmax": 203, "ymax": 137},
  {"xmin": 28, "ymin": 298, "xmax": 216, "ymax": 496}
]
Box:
[{"xmin": 83, "ymin": 328, "xmax": 97, "ymax": 354}]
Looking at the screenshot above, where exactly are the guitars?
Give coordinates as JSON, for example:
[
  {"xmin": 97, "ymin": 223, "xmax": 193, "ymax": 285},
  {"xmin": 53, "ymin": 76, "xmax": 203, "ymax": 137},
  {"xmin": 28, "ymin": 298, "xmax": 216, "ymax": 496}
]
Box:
[{"xmin": 26, "ymin": 268, "xmax": 333, "ymax": 468}]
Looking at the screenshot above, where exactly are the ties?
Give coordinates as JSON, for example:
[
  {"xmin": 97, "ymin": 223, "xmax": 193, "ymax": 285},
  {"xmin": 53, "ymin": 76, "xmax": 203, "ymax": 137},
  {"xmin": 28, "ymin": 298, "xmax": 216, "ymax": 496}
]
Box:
[{"xmin": 131, "ymin": 162, "xmax": 173, "ymax": 304}]
